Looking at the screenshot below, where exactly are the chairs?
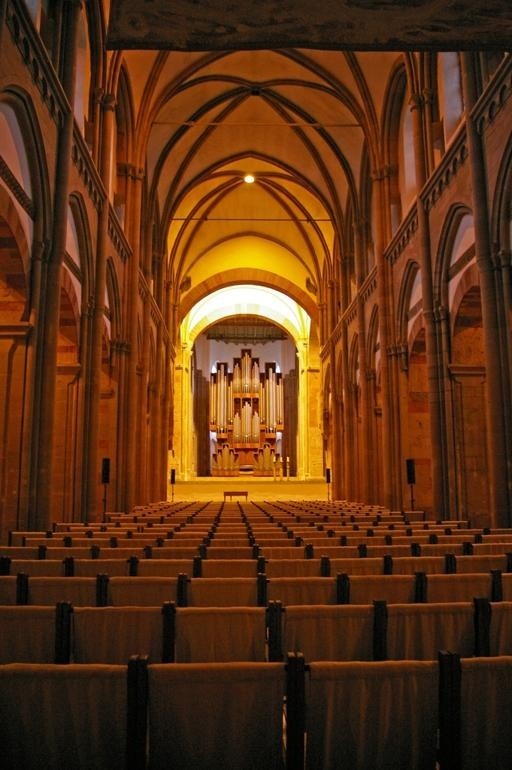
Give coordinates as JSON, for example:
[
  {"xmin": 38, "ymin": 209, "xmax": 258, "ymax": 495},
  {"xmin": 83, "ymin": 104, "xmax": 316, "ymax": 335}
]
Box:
[{"xmin": 3, "ymin": 502, "xmax": 510, "ymax": 769}]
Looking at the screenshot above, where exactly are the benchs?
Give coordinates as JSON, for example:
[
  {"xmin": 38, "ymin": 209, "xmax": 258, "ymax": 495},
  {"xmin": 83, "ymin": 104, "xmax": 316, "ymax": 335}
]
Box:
[{"xmin": 222, "ymin": 491, "xmax": 248, "ymax": 501}]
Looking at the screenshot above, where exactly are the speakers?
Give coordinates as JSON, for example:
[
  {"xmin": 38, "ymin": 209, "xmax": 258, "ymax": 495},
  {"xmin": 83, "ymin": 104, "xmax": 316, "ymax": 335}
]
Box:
[
  {"xmin": 100, "ymin": 457, "xmax": 110, "ymax": 484},
  {"xmin": 405, "ymin": 458, "xmax": 416, "ymax": 484},
  {"xmin": 170, "ymin": 469, "xmax": 175, "ymax": 484},
  {"xmin": 326, "ymin": 467, "xmax": 331, "ymax": 484}
]
